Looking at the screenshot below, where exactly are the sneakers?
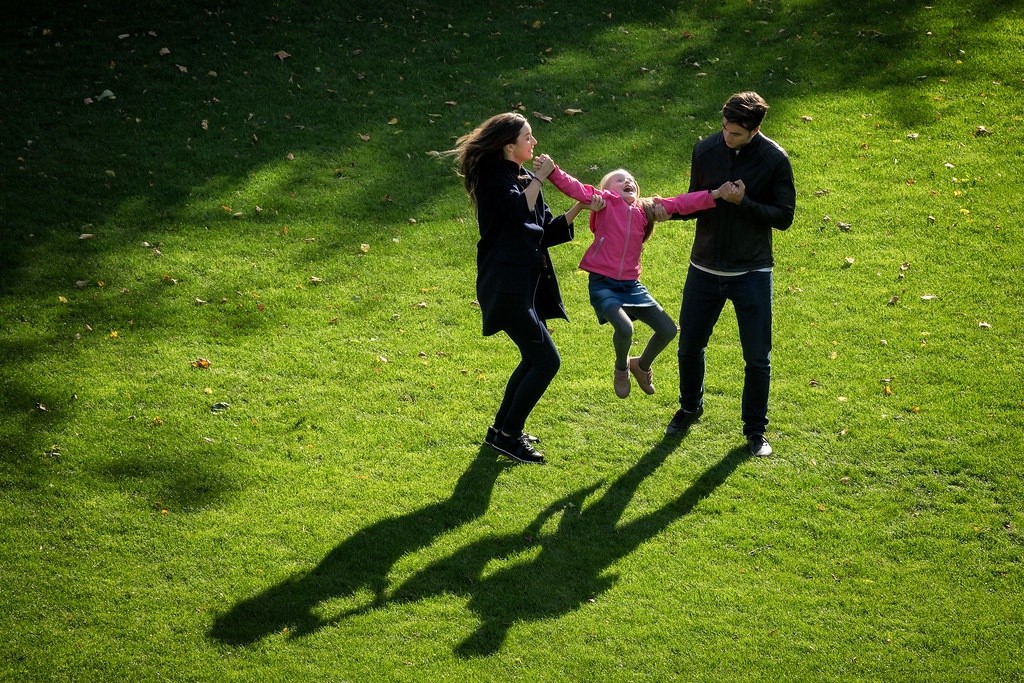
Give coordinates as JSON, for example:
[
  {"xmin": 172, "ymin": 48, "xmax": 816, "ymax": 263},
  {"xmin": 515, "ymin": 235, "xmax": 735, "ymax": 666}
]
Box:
[
  {"xmin": 492, "ymin": 428, "xmax": 545, "ymax": 463},
  {"xmin": 484, "ymin": 426, "xmax": 540, "ymax": 444}
]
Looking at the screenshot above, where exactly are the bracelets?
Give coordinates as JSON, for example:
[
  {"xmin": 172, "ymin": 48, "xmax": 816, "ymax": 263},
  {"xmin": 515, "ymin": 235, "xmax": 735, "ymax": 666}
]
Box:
[{"xmin": 532, "ymin": 176, "xmax": 543, "ymax": 186}]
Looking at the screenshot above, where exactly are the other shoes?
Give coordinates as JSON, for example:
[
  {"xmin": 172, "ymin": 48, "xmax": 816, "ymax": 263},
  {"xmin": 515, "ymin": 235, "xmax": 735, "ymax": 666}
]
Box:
[
  {"xmin": 747, "ymin": 432, "xmax": 773, "ymax": 457},
  {"xmin": 666, "ymin": 402, "xmax": 703, "ymax": 437},
  {"xmin": 614, "ymin": 366, "xmax": 631, "ymax": 399},
  {"xmin": 630, "ymin": 358, "xmax": 655, "ymax": 395}
]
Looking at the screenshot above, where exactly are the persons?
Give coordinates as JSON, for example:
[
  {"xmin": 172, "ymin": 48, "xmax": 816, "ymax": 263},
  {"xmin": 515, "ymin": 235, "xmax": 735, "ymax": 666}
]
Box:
[
  {"xmin": 534, "ymin": 152, "xmax": 740, "ymax": 400},
  {"xmin": 453, "ymin": 111, "xmax": 607, "ymax": 465},
  {"xmin": 654, "ymin": 92, "xmax": 795, "ymax": 457}
]
